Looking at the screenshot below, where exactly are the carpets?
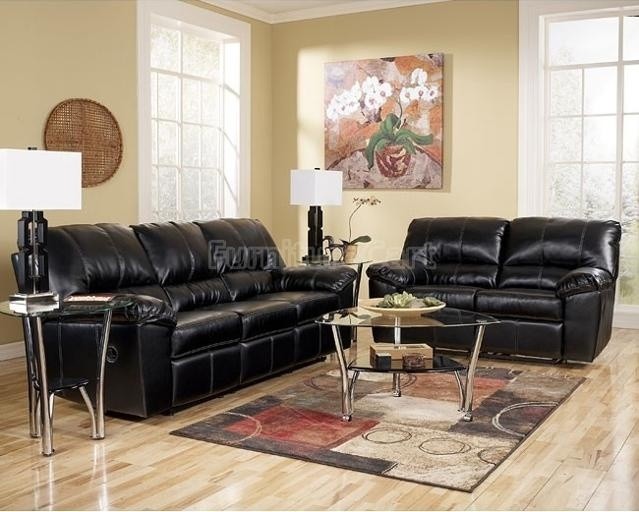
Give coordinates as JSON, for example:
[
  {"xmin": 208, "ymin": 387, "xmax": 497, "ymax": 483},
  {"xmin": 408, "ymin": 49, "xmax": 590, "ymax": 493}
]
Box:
[{"xmin": 169, "ymin": 354, "xmax": 592, "ymax": 494}]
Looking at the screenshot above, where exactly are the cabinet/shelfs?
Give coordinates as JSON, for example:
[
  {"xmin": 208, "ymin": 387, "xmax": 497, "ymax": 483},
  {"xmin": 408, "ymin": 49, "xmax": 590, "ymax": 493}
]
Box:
[{"xmin": 311, "ymin": 305, "xmax": 504, "ymax": 429}]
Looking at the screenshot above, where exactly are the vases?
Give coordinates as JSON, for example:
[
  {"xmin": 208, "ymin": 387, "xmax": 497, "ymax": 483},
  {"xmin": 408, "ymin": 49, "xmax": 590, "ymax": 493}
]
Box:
[{"xmin": 340, "ymin": 245, "xmax": 358, "ymax": 263}]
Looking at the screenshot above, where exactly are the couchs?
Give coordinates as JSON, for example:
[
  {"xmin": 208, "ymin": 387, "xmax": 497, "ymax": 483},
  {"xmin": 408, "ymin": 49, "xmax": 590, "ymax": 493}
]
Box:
[
  {"xmin": 10, "ymin": 214, "xmax": 359, "ymax": 426},
  {"xmin": 366, "ymin": 215, "xmax": 623, "ymax": 364}
]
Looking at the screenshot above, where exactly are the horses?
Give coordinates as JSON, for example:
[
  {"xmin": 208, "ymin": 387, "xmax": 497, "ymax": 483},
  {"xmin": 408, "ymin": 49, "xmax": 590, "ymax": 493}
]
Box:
[{"xmin": 322, "ymin": 235, "xmax": 347, "ymax": 263}]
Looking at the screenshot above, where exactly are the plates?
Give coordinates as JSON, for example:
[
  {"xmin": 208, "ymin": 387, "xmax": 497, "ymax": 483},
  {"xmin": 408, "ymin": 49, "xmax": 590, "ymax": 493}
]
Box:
[
  {"xmin": 359, "ymin": 297, "xmax": 446, "ymax": 318},
  {"xmin": 359, "ymin": 316, "xmax": 444, "ymax": 326}
]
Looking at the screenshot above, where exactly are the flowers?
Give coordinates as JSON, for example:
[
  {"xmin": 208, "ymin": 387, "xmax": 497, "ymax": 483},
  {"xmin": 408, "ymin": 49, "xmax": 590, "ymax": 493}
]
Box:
[{"xmin": 332, "ymin": 193, "xmax": 383, "ymax": 247}]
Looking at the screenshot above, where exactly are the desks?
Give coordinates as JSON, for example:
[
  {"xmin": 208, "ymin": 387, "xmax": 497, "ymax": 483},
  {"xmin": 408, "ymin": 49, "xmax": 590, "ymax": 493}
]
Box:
[
  {"xmin": 303, "ymin": 255, "xmax": 374, "ymax": 348},
  {"xmin": 0, "ymin": 291, "xmax": 138, "ymax": 456}
]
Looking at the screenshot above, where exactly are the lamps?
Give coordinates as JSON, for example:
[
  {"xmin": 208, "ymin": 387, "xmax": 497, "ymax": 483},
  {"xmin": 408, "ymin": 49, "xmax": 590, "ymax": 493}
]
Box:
[
  {"xmin": 288, "ymin": 166, "xmax": 344, "ymax": 261},
  {"xmin": 0, "ymin": 142, "xmax": 83, "ymax": 310}
]
